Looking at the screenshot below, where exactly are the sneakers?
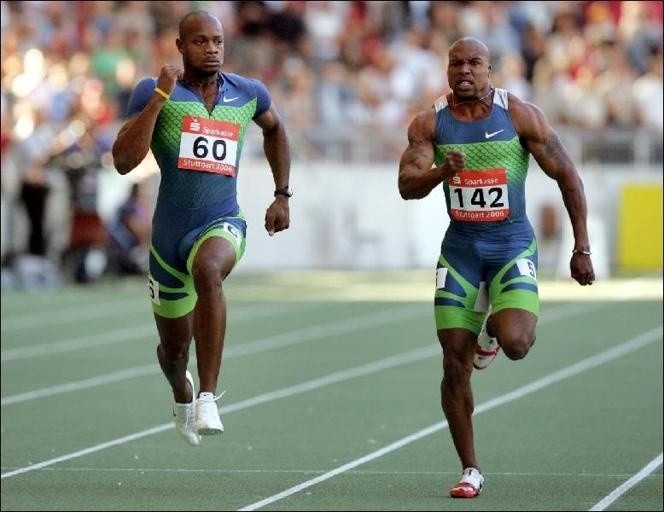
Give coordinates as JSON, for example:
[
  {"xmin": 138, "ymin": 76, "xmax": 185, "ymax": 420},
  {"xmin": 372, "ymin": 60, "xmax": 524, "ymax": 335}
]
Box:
[
  {"xmin": 169, "ymin": 369, "xmax": 202, "ymax": 446},
  {"xmin": 472, "ymin": 322, "xmax": 501, "ymax": 370},
  {"xmin": 449, "ymin": 466, "xmax": 485, "ymax": 499},
  {"xmin": 193, "ymin": 392, "xmax": 224, "ymax": 436}
]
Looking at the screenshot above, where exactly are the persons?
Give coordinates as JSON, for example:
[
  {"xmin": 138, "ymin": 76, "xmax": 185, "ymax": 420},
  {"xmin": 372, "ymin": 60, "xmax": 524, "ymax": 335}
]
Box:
[
  {"xmin": 111, "ymin": 7, "xmax": 294, "ymax": 446},
  {"xmin": 396, "ymin": 32, "xmax": 597, "ymax": 499},
  {"xmin": 1, "ymin": 1, "xmax": 664, "ymax": 290}
]
